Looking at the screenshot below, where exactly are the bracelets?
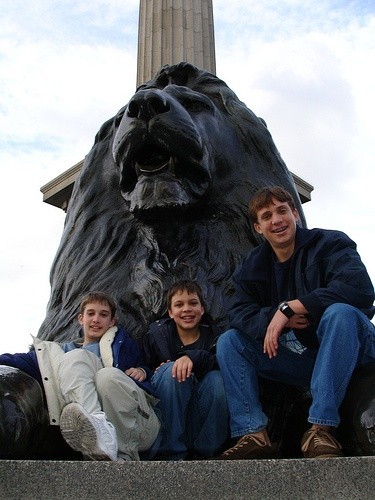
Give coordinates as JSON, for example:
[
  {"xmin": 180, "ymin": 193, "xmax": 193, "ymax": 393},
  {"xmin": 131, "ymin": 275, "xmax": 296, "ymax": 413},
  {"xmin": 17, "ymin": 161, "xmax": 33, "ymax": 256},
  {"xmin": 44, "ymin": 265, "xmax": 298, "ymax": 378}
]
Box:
[{"xmin": 278, "ymin": 301, "xmax": 295, "ymax": 319}]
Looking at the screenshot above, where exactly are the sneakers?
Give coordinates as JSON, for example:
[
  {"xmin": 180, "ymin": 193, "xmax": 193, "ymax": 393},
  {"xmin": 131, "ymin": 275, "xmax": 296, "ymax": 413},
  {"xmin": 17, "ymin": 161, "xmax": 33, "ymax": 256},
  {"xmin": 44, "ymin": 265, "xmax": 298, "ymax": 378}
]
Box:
[
  {"xmin": 59, "ymin": 402, "xmax": 119, "ymax": 462},
  {"xmin": 116, "ymin": 451, "xmax": 141, "ymax": 461}
]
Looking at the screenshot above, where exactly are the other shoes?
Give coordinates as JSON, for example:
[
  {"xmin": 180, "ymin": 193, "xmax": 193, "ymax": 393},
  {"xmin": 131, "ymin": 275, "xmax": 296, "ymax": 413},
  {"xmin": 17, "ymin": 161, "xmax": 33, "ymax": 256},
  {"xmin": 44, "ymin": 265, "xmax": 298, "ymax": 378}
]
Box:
[
  {"xmin": 299, "ymin": 427, "xmax": 345, "ymax": 458},
  {"xmin": 219, "ymin": 433, "xmax": 282, "ymax": 460}
]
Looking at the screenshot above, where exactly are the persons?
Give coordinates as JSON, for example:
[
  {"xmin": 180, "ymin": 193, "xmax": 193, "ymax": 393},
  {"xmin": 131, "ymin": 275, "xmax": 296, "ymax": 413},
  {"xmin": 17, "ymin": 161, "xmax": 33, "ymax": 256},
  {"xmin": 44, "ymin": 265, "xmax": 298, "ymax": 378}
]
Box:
[
  {"xmin": 216, "ymin": 185, "xmax": 375, "ymax": 460},
  {"xmin": 143, "ymin": 279, "xmax": 231, "ymax": 461},
  {"xmin": 0, "ymin": 291, "xmax": 166, "ymax": 462}
]
